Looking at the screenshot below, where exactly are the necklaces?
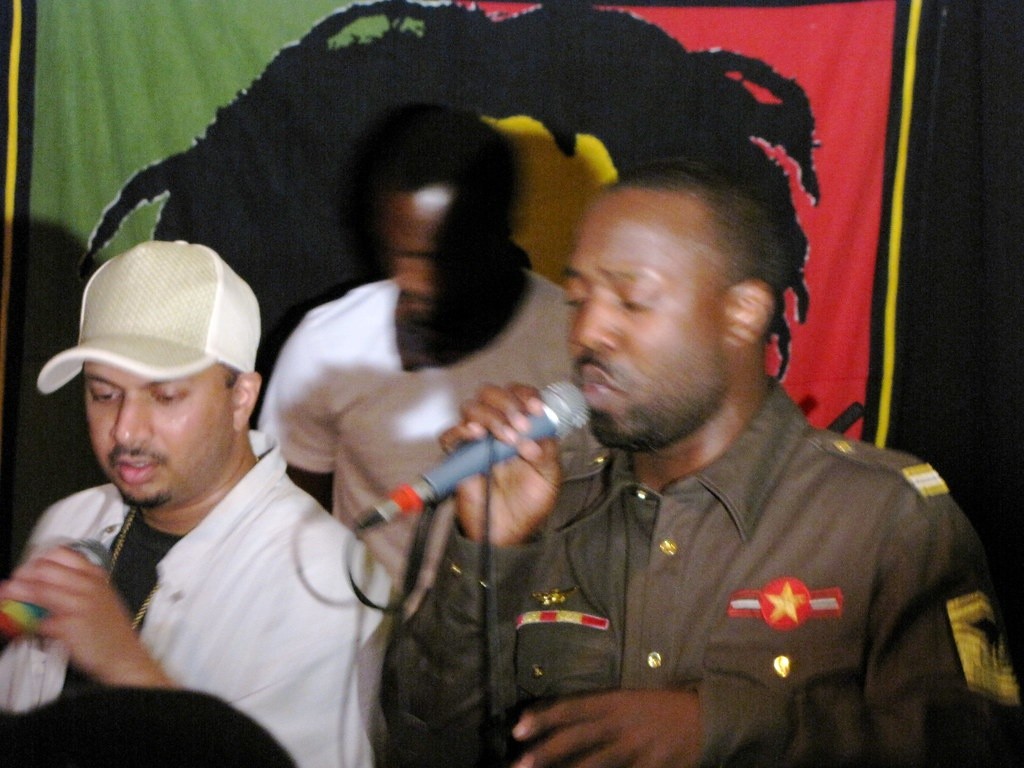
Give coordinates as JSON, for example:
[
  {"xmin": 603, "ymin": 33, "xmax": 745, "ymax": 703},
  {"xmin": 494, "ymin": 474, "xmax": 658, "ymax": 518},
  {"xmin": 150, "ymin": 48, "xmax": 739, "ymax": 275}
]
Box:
[{"xmin": 104, "ymin": 506, "xmax": 160, "ymax": 632}]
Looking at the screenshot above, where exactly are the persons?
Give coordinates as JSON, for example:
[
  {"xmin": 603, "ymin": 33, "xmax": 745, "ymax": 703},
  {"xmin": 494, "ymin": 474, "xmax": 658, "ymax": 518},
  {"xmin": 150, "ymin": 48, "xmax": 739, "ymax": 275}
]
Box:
[
  {"xmin": 256, "ymin": 109, "xmax": 600, "ymax": 621},
  {"xmin": 378, "ymin": 154, "xmax": 1024, "ymax": 768},
  {"xmin": 0, "ymin": 240, "xmax": 393, "ymax": 768}
]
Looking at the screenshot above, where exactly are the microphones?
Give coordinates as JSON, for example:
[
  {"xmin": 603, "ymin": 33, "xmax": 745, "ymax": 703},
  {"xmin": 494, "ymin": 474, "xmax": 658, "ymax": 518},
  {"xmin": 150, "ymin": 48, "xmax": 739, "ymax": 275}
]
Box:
[
  {"xmin": 357, "ymin": 380, "xmax": 589, "ymax": 534},
  {"xmin": 0, "ymin": 539, "xmax": 113, "ymax": 649}
]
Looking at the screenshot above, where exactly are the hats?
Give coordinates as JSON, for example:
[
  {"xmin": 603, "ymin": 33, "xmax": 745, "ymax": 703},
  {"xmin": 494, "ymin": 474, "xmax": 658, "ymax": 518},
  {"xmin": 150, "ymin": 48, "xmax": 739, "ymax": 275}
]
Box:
[{"xmin": 37, "ymin": 239, "xmax": 262, "ymax": 395}]
